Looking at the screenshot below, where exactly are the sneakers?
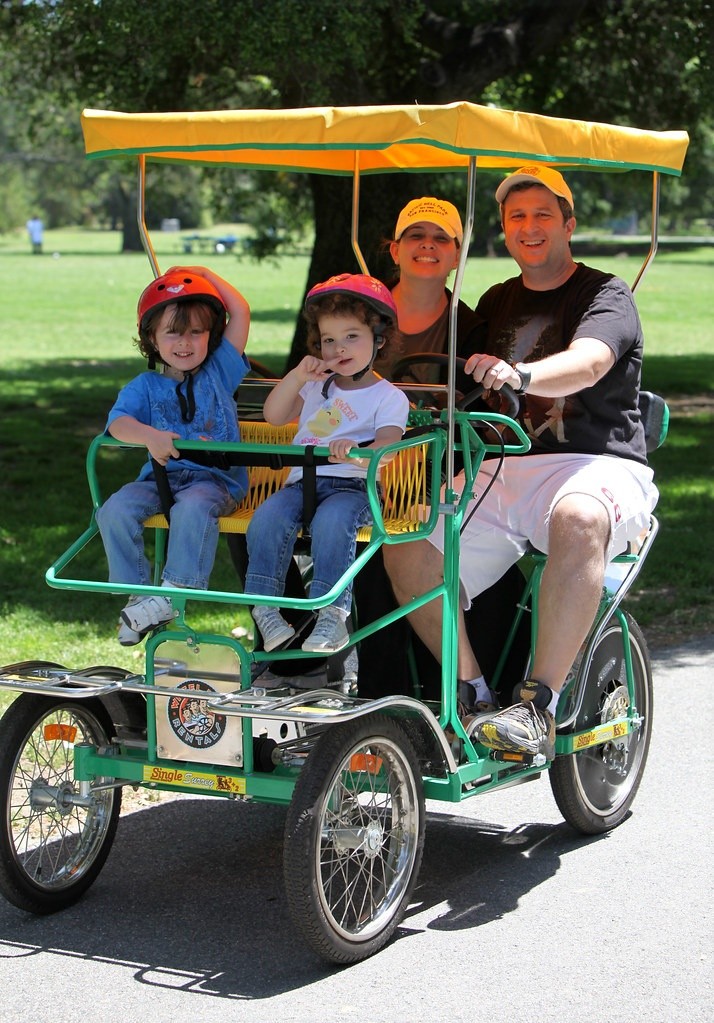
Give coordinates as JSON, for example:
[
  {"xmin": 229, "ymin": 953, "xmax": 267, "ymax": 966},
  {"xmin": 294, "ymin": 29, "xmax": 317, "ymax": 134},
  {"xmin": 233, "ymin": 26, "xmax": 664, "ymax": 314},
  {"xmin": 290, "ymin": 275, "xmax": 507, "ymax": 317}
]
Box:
[
  {"xmin": 477, "ymin": 679, "xmax": 556, "ymax": 761},
  {"xmin": 301, "ymin": 605, "xmax": 350, "ymax": 654},
  {"xmin": 250, "ymin": 605, "xmax": 295, "ymax": 653},
  {"xmin": 117, "ymin": 595, "xmax": 173, "ymax": 647},
  {"xmin": 444, "ymin": 678, "xmax": 500, "ymax": 744}
]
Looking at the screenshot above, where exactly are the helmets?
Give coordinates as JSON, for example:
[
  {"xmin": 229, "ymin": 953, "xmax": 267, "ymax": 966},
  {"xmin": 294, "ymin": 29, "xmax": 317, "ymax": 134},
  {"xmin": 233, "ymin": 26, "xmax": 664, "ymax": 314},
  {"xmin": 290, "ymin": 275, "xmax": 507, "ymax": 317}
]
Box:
[
  {"xmin": 136, "ymin": 271, "xmax": 227, "ymax": 341},
  {"xmin": 304, "ymin": 273, "xmax": 399, "ymax": 333}
]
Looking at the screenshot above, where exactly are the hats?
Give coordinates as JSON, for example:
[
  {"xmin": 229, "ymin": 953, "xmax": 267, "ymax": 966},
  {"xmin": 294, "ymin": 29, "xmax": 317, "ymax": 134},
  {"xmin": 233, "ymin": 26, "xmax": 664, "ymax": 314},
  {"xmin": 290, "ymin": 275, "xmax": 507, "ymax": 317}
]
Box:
[
  {"xmin": 394, "ymin": 197, "xmax": 463, "ymax": 246},
  {"xmin": 494, "ymin": 165, "xmax": 574, "ymax": 213}
]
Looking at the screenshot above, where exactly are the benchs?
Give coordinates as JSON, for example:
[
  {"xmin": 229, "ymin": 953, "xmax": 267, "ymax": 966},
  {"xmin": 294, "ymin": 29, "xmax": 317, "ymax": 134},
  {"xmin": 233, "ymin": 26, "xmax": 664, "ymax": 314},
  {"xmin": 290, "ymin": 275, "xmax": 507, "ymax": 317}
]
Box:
[{"xmin": 140, "ymin": 418, "xmax": 434, "ymax": 551}]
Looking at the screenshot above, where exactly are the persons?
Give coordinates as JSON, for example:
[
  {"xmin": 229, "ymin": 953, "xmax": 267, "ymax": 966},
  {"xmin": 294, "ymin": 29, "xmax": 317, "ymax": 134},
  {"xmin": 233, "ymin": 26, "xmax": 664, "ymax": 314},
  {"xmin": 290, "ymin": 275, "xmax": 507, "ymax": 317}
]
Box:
[
  {"xmin": 27, "ymin": 214, "xmax": 46, "ymax": 255},
  {"xmin": 239, "ymin": 270, "xmax": 410, "ymax": 655},
  {"xmin": 92, "ymin": 264, "xmax": 255, "ymax": 647},
  {"xmin": 386, "ymin": 164, "xmax": 660, "ymax": 757},
  {"xmin": 225, "ymin": 197, "xmax": 485, "ymax": 690}
]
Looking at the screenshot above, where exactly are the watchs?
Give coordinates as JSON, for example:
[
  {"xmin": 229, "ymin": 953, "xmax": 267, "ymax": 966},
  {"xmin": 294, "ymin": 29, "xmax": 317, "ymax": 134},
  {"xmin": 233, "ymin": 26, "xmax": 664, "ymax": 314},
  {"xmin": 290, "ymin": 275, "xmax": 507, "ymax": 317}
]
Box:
[{"xmin": 510, "ymin": 361, "xmax": 531, "ymax": 396}]
